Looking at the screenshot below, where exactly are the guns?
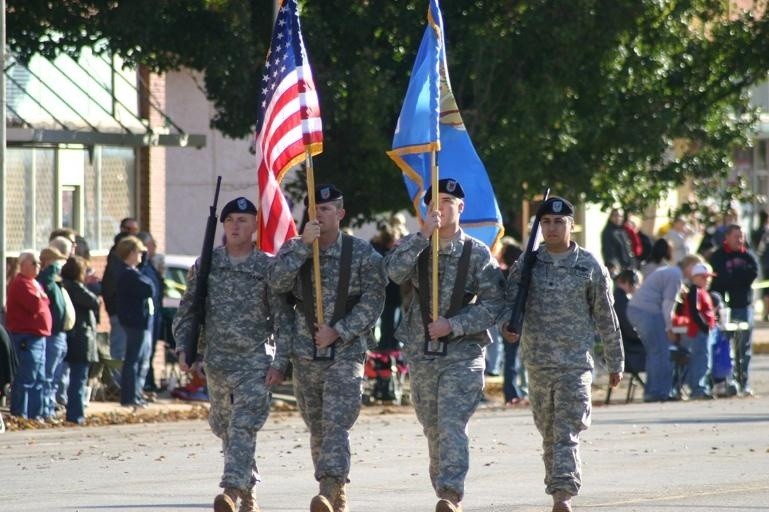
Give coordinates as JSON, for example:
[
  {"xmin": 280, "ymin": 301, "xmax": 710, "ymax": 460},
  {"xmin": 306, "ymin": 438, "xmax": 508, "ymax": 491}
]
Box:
[
  {"xmin": 186, "ymin": 176, "xmax": 222, "ymax": 364},
  {"xmin": 507, "ymin": 188, "xmax": 550, "ymax": 334}
]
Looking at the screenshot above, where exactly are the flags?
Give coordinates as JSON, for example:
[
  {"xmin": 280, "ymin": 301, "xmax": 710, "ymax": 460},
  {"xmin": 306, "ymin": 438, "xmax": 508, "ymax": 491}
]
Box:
[
  {"xmin": 253, "ymin": 1, "xmax": 324, "ymax": 259},
  {"xmin": 384, "ymin": 1, "xmax": 506, "ymax": 261}
]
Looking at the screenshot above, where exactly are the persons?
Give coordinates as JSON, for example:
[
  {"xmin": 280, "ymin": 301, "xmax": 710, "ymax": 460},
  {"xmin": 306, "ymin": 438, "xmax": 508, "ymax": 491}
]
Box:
[
  {"xmin": 264, "ymin": 182, "xmax": 387, "ymax": 512},
  {"xmin": 497, "ymin": 196, "xmax": 625, "ymax": 512},
  {"xmin": 171, "ymin": 197, "xmax": 295, "ymax": 511},
  {"xmin": 601, "ymin": 203, "xmax": 769, "ymax": 401},
  {"xmin": 382, "ymin": 177, "xmax": 506, "ymax": 512},
  {"xmin": 172, "ymin": 223, "xmax": 532, "ymax": 404},
  {"xmin": 4, "ymin": 218, "xmax": 167, "ymax": 426}
]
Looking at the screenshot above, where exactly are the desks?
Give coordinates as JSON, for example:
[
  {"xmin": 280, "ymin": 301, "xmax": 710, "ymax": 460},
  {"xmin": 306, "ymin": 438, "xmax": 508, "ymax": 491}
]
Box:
[{"xmin": 669, "ymin": 318, "xmax": 751, "ymax": 398}]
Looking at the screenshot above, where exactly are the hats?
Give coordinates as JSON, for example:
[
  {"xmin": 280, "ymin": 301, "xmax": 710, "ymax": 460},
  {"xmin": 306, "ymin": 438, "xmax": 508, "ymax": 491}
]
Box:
[
  {"xmin": 40, "ymin": 247, "xmax": 69, "ymax": 260},
  {"xmin": 423, "ymin": 177, "xmax": 466, "ymax": 206},
  {"xmin": 304, "ymin": 185, "xmax": 344, "ymax": 204},
  {"xmin": 219, "ymin": 196, "xmax": 258, "ymax": 224},
  {"xmin": 536, "ymin": 196, "xmax": 575, "ymax": 222},
  {"xmin": 691, "ymin": 262, "xmax": 717, "ymax": 278}
]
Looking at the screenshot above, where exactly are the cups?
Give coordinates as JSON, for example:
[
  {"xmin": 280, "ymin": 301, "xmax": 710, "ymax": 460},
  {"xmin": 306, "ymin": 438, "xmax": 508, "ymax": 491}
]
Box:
[{"xmin": 717, "ymin": 304, "xmax": 733, "ymax": 323}]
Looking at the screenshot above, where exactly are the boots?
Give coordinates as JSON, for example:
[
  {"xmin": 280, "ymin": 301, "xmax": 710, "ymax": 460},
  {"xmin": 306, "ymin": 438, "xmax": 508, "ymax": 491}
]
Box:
[
  {"xmin": 550, "ymin": 492, "xmax": 573, "ymax": 512},
  {"xmin": 239, "ymin": 485, "xmax": 259, "ymax": 511},
  {"xmin": 213, "ymin": 485, "xmax": 239, "ymax": 511},
  {"xmin": 436, "ymin": 494, "xmax": 462, "ymax": 512},
  {"xmin": 309, "ymin": 475, "xmax": 347, "ymax": 512}
]
